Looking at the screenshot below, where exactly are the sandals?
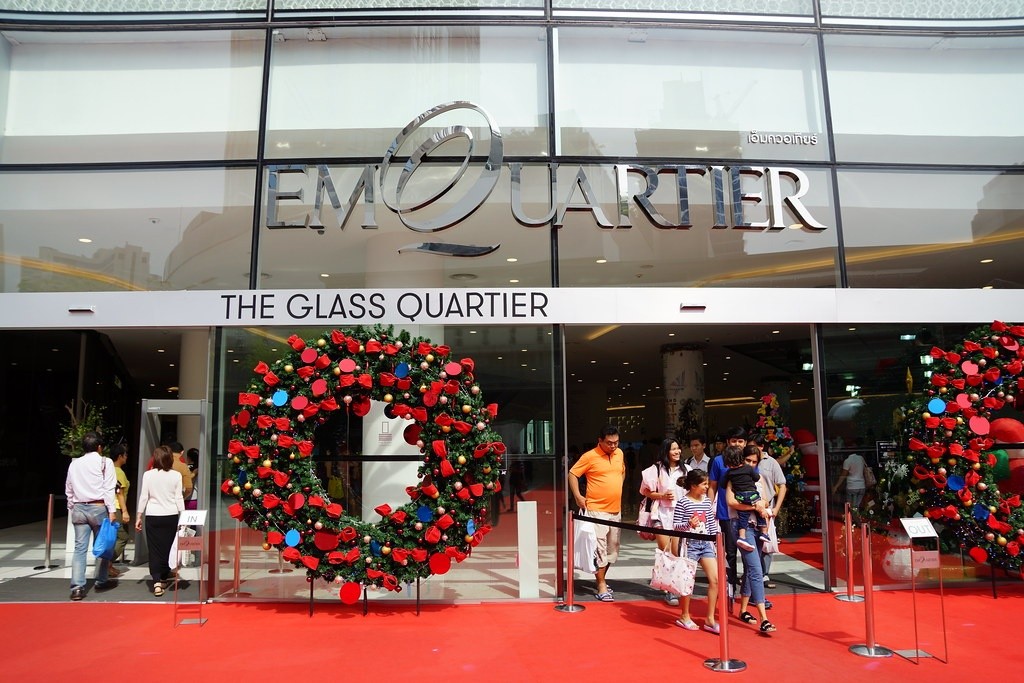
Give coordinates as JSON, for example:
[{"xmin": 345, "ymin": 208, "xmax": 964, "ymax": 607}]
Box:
[
  {"xmin": 153, "ymin": 586, "xmax": 165, "ymax": 595},
  {"xmin": 160, "ymin": 580, "xmax": 169, "ymax": 589},
  {"xmin": 763, "ymin": 580, "xmax": 776, "ymax": 589}
]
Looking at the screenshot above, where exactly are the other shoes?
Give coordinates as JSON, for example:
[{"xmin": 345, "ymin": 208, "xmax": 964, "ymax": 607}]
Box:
[
  {"xmin": 70, "ymin": 588, "xmax": 84, "ymax": 600},
  {"xmin": 759, "ymin": 533, "xmax": 771, "ymax": 543},
  {"xmin": 108, "ymin": 566, "xmax": 120, "ymax": 576},
  {"xmin": 95, "ymin": 578, "xmax": 119, "ymax": 592},
  {"xmin": 508, "ymin": 509, "xmax": 514, "ymax": 511},
  {"xmin": 664, "ymin": 592, "xmax": 680, "ymax": 605},
  {"xmin": 737, "ymin": 537, "xmax": 755, "ymax": 552}
]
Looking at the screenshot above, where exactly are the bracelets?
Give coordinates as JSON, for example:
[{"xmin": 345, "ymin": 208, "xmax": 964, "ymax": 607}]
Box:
[{"xmin": 788, "ymin": 452, "xmax": 792, "ymax": 456}]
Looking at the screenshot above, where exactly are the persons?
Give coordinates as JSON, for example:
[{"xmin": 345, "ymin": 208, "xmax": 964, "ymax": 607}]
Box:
[
  {"xmin": 109, "ymin": 445, "xmax": 131, "ymax": 577},
  {"xmin": 833, "ymin": 445, "xmax": 866, "ymax": 528},
  {"xmin": 65, "ymin": 430, "xmax": 119, "ymax": 600},
  {"xmin": 135, "ymin": 442, "xmax": 199, "ymax": 595},
  {"xmin": 639, "ymin": 425, "xmax": 796, "ymax": 632},
  {"xmin": 569, "ymin": 428, "xmax": 625, "ymax": 601},
  {"xmin": 327, "ymin": 468, "xmax": 344, "ymax": 502}
]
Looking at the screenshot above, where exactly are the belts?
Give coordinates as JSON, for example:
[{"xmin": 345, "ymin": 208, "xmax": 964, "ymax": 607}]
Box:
[{"xmin": 83, "ymin": 499, "xmax": 104, "ymax": 504}]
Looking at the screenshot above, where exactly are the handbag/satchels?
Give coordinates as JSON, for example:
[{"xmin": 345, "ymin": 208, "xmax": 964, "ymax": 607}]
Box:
[
  {"xmin": 574, "ymin": 509, "xmax": 599, "ymax": 574},
  {"xmin": 92, "ymin": 518, "xmax": 121, "ymax": 559},
  {"xmin": 637, "ymin": 497, "xmax": 657, "ymax": 542},
  {"xmin": 858, "ymin": 455, "xmax": 878, "ymax": 487},
  {"xmin": 168, "ymin": 526, "xmax": 197, "ymax": 568},
  {"xmin": 762, "ymin": 518, "xmax": 779, "ymax": 553},
  {"xmin": 650, "ymin": 533, "xmax": 698, "ymax": 598}
]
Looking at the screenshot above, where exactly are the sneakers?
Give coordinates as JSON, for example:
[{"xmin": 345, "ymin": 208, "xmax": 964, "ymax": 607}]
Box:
[{"xmin": 748, "ymin": 596, "xmax": 772, "ymax": 608}]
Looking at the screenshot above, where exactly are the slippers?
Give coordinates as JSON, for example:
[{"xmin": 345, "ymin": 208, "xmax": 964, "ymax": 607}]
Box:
[
  {"xmin": 739, "ymin": 610, "xmax": 757, "ymax": 624},
  {"xmin": 704, "ymin": 623, "xmax": 719, "ymax": 633},
  {"xmin": 760, "ymin": 620, "xmax": 777, "ymax": 632},
  {"xmin": 676, "ymin": 617, "xmax": 700, "ymax": 631},
  {"xmin": 606, "ymin": 584, "xmax": 614, "ymax": 594},
  {"xmin": 595, "ymin": 592, "xmax": 615, "ymax": 601}
]
